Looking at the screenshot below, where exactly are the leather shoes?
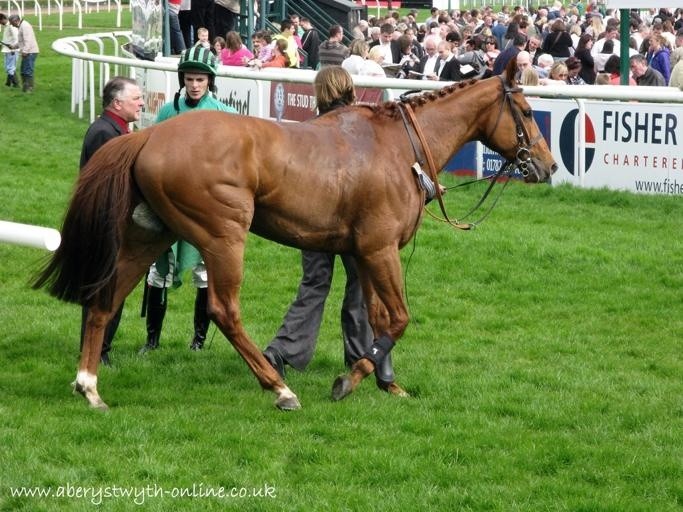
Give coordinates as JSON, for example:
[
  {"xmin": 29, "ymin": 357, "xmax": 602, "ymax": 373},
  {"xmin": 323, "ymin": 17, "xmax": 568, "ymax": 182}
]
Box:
[{"xmin": 264, "ymin": 347, "xmax": 287, "ymax": 381}]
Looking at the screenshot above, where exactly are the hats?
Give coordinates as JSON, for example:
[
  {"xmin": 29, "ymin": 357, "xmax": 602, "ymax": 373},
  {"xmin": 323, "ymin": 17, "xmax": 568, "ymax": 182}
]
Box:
[{"xmin": 177, "ymin": 47, "xmax": 217, "ymax": 90}]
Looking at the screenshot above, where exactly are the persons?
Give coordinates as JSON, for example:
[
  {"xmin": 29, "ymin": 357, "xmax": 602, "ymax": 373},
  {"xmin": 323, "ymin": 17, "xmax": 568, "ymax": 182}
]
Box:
[
  {"xmin": 138, "ymin": 43, "xmax": 242, "ymax": 356},
  {"xmin": 78, "ymin": 73, "xmax": 146, "ymax": 366},
  {"xmin": 167, "ymin": 0, "xmax": 683, "ymax": 104},
  {"xmin": 259, "ymin": 64, "xmax": 447, "ymax": 383},
  {"xmin": 0, "ymin": 14, "xmax": 18, "ymax": 88},
  {"xmin": 5, "ymin": 14, "xmax": 40, "ymax": 93}
]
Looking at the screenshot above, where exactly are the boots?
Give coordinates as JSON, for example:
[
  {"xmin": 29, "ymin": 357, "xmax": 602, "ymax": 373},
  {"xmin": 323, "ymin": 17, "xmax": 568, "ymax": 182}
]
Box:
[
  {"xmin": 6, "ymin": 73, "xmax": 34, "ymax": 94},
  {"xmin": 191, "ymin": 284, "xmax": 212, "ymax": 353},
  {"xmin": 139, "ymin": 284, "xmax": 171, "ymax": 354}
]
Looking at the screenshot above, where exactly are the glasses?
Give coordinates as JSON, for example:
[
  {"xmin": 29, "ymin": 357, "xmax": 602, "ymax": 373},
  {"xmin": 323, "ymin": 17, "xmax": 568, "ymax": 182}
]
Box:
[{"xmin": 486, "ymin": 39, "xmax": 495, "ymax": 45}]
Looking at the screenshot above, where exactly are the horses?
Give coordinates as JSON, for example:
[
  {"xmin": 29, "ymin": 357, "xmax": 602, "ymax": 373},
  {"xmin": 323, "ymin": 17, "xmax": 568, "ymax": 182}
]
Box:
[{"xmin": 25, "ymin": 52, "xmax": 559, "ymax": 413}]
